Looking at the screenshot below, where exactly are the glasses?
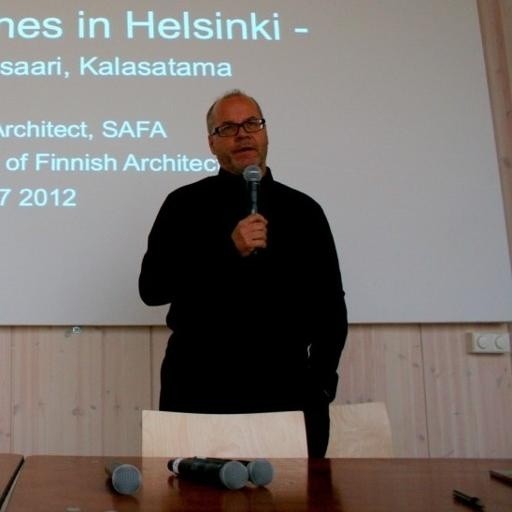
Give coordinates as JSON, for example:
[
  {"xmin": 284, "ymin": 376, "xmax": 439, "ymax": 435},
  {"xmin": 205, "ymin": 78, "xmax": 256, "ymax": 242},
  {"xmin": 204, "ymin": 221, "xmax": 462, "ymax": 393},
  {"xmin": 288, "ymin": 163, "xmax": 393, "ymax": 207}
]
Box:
[{"xmin": 212, "ymin": 118, "xmax": 265, "ymax": 137}]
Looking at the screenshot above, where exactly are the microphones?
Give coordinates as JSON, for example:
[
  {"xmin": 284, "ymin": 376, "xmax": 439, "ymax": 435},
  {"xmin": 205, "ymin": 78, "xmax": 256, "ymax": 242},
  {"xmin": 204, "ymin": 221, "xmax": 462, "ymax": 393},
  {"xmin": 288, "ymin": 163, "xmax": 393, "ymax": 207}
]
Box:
[
  {"xmin": 105, "ymin": 461, "xmax": 141, "ymax": 495},
  {"xmin": 243, "ymin": 164, "xmax": 263, "ymax": 254},
  {"xmin": 195, "ymin": 458, "xmax": 274, "ymax": 486},
  {"xmin": 168, "ymin": 457, "xmax": 248, "ymax": 491}
]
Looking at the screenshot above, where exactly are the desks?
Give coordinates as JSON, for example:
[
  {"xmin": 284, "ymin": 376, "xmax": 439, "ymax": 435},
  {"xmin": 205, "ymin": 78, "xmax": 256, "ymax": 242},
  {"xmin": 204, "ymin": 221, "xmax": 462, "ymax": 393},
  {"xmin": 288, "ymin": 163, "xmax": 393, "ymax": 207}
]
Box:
[
  {"xmin": 0, "ymin": 453, "xmax": 512, "ymax": 512},
  {"xmin": 1, "ymin": 453, "xmax": 23, "ymax": 503}
]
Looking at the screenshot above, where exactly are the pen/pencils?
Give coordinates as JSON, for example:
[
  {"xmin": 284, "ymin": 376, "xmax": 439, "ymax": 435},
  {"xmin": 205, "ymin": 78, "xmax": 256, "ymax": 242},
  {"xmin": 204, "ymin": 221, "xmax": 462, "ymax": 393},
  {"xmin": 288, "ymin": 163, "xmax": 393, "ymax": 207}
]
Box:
[{"xmin": 454, "ymin": 489, "xmax": 480, "ymax": 502}]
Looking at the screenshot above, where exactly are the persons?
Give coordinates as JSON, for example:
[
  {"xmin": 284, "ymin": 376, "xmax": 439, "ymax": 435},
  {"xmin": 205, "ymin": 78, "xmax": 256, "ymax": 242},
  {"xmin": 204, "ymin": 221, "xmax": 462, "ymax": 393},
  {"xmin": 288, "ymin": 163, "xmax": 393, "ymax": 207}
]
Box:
[{"xmin": 138, "ymin": 90, "xmax": 348, "ymax": 459}]
